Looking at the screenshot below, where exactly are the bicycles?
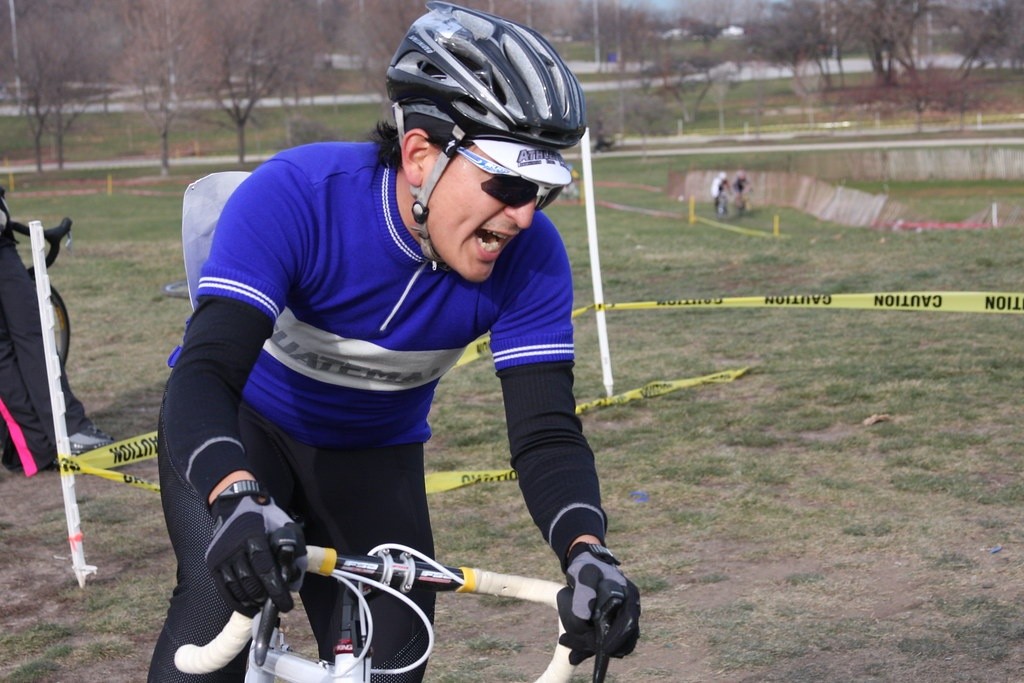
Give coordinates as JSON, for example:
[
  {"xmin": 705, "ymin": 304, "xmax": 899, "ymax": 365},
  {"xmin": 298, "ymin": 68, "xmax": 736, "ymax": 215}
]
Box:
[
  {"xmin": 10, "ymin": 217, "xmax": 73, "ymax": 365},
  {"xmin": 172, "ymin": 535, "xmax": 624, "ymax": 683}
]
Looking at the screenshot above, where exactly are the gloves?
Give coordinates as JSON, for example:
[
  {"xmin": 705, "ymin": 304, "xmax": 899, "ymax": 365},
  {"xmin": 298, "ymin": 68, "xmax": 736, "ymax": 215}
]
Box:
[
  {"xmin": 559, "ymin": 541, "xmax": 640, "ymax": 666},
  {"xmin": 203, "ymin": 479, "xmax": 309, "ymax": 615}
]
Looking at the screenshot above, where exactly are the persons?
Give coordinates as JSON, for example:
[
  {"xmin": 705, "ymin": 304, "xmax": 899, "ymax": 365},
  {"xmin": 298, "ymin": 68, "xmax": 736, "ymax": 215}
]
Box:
[
  {"xmin": 0, "ymin": 188, "xmax": 117, "ymax": 477},
  {"xmin": 593, "ymin": 110, "xmax": 610, "ymax": 152},
  {"xmin": 144, "ymin": 0, "xmax": 642, "ymax": 683},
  {"xmin": 733, "ymin": 168, "xmax": 754, "ymax": 203},
  {"xmin": 709, "ymin": 171, "xmax": 733, "ymax": 211}
]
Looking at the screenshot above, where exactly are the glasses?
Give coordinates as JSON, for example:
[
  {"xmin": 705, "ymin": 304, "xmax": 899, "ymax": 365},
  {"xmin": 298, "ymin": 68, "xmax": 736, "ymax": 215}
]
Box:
[{"xmin": 455, "ymin": 145, "xmax": 564, "ymax": 213}]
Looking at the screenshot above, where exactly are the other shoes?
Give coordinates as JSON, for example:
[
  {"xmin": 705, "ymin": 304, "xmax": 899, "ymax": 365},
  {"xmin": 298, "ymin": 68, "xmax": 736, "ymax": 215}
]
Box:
[{"xmin": 66, "ymin": 425, "xmax": 123, "ymax": 456}]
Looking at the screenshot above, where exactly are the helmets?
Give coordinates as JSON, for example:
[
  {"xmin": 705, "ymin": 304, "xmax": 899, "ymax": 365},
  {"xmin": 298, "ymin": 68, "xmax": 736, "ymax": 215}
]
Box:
[
  {"xmin": 718, "ymin": 171, "xmax": 728, "ymax": 179},
  {"xmin": 385, "ymin": 1, "xmax": 588, "ymax": 151}
]
